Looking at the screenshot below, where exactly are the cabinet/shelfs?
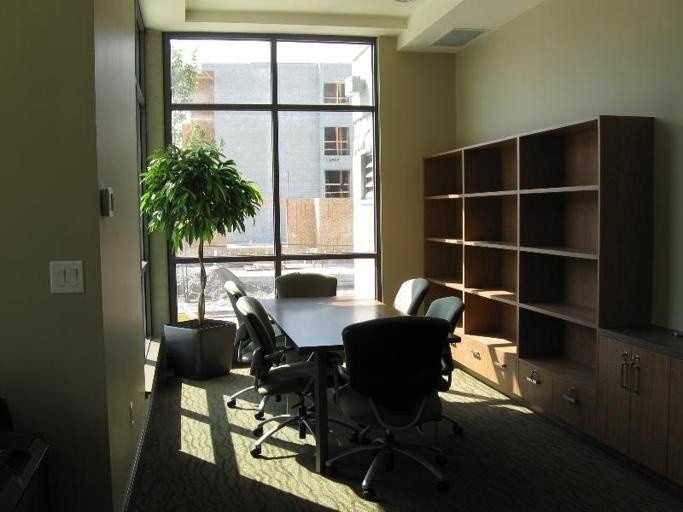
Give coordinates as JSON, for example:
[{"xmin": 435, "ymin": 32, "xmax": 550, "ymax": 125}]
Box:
[{"xmin": 421, "ymin": 114, "xmax": 682, "ymax": 497}]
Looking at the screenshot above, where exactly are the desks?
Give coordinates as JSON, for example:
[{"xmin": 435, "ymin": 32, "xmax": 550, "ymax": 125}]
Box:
[{"xmin": 257, "ymin": 294, "xmax": 408, "ymax": 475}]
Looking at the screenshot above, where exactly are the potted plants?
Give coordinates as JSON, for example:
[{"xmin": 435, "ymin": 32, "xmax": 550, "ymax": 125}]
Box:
[{"xmin": 138, "ymin": 143, "xmax": 263, "ymax": 381}]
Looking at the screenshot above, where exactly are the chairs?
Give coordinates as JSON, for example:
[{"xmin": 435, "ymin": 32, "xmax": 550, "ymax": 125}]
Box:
[
  {"xmin": 392, "ymin": 278, "xmax": 429, "ymax": 317},
  {"xmin": 423, "ymin": 296, "xmax": 464, "ymax": 433},
  {"xmin": 324, "ymin": 315, "xmax": 463, "ymax": 497},
  {"xmin": 227, "ymin": 296, "xmax": 312, "ymax": 418},
  {"xmin": 223, "ymin": 280, "xmax": 283, "ymax": 364},
  {"xmin": 276, "ymin": 273, "xmax": 337, "ymax": 298}
]
